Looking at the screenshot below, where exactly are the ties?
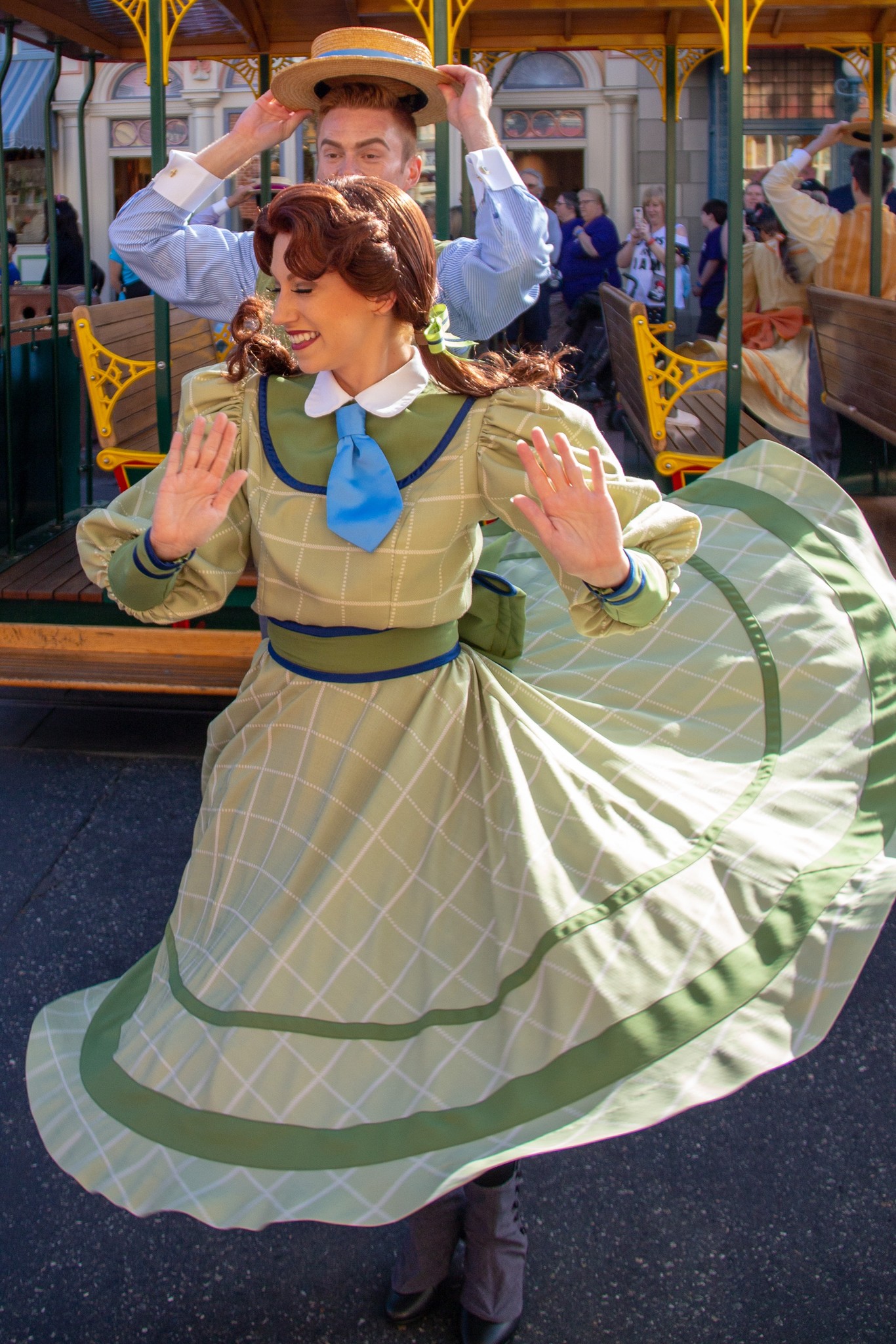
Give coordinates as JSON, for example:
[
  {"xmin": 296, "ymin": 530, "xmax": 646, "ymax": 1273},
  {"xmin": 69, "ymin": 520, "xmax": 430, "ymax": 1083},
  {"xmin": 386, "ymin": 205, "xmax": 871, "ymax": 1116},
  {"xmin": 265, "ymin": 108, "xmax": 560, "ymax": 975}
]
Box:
[{"xmin": 325, "ymin": 403, "xmax": 406, "ymax": 555}]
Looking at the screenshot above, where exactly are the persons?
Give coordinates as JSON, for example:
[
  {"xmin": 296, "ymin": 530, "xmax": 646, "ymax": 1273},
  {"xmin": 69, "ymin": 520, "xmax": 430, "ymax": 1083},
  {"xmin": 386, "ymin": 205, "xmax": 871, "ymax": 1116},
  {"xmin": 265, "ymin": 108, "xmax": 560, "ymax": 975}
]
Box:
[
  {"xmin": 41, "ymin": 194, "xmax": 87, "ymax": 286},
  {"xmin": 105, "ymin": 62, "xmax": 552, "ymax": 360},
  {"xmin": 66, "ymin": 259, "xmax": 105, "ymax": 309},
  {"xmin": 690, "ymin": 197, "xmax": 731, "ymax": 346},
  {"xmin": 756, "ymin": 119, "xmax": 896, "ymax": 482},
  {"xmin": 495, "ymin": 167, "xmax": 563, "ymax": 352},
  {"xmin": 562, "ymin": 186, "xmax": 623, "ymax": 403},
  {"xmin": 542, "ymin": 193, "xmax": 586, "ymax": 354},
  {"xmin": 6, "ymin": 226, "xmax": 21, "ymax": 286},
  {"xmin": 108, "ymin": 246, "xmax": 156, "ymax": 304},
  {"xmin": 614, "ymin": 181, "xmax": 691, "ymax": 371},
  {"xmin": 74, "ymin": 166, "xmax": 706, "ymax": 1343},
  {"xmin": 715, "ymin": 198, "xmax": 811, "ymax": 438},
  {"xmin": 720, "ymin": 180, "xmax": 772, "ymax": 263},
  {"xmin": 827, "ymin": 146, "xmax": 896, "ymax": 217},
  {"xmin": 188, "ymin": 175, "xmax": 296, "ymax": 232}
]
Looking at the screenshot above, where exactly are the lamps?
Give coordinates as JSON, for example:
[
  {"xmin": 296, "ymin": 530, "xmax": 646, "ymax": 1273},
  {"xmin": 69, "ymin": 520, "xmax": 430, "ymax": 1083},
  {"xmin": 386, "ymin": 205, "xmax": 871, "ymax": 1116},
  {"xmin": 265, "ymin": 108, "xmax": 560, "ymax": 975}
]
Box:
[{"xmin": 833, "ymin": 51, "xmax": 869, "ymax": 103}]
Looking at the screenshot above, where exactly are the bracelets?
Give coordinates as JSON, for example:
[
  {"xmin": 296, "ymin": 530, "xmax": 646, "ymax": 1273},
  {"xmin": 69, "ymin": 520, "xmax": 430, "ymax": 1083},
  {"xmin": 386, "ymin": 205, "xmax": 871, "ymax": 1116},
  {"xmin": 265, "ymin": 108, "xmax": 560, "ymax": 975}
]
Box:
[
  {"xmin": 576, "ymin": 230, "xmax": 585, "ymax": 235},
  {"xmin": 644, "ymin": 238, "xmax": 656, "ymax": 247}
]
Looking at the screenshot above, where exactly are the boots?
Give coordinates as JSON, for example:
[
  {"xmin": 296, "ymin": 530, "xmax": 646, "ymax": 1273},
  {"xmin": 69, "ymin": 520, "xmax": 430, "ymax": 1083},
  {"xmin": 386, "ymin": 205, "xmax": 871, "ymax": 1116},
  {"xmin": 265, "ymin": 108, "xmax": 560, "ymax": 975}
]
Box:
[
  {"xmin": 379, "ymin": 1183, "xmax": 465, "ymax": 1318},
  {"xmin": 462, "ymin": 1158, "xmax": 527, "ymax": 1343}
]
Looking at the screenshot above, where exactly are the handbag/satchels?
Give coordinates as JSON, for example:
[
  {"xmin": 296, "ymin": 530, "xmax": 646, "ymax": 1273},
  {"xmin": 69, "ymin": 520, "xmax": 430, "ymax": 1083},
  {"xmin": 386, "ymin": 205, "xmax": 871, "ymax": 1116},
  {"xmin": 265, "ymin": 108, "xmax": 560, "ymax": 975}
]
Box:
[{"xmin": 122, "ymin": 278, "xmax": 151, "ymax": 299}]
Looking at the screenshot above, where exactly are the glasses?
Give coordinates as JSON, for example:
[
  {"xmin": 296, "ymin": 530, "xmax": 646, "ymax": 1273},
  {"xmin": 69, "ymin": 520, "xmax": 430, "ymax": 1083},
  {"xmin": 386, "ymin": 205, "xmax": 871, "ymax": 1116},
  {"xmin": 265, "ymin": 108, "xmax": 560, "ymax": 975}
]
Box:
[
  {"xmin": 555, "ymin": 201, "xmax": 570, "ymax": 206},
  {"xmin": 578, "ymin": 200, "xmax": 598, "ymax": 204}
]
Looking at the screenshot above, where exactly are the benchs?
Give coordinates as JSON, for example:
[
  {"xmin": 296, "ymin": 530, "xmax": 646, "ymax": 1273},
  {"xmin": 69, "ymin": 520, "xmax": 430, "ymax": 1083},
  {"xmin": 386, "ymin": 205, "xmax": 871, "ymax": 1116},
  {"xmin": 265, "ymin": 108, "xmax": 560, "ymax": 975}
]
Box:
[
  {"xmin": 600, "ymin": 281, "xmax": 781, "ymax": 495},
  {"xmin": 71, "ymin": 294, "xmax": 235, "ymax": 493},
  {"xmin": 806, "ymin": 281, "xmax": 896, "ymax": 448}
]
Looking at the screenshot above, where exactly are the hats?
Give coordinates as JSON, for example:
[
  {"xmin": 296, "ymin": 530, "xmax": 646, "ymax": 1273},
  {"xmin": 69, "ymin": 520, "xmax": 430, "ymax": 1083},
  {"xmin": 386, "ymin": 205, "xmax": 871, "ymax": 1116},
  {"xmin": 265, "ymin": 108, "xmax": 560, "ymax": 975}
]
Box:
[
  {"xmin": 272, "ymin": 27, "xmax": 466, "ymax": 128},
  {"xmin": 841, "ymin": 109, "xmax": 896, "ymax": 149}
]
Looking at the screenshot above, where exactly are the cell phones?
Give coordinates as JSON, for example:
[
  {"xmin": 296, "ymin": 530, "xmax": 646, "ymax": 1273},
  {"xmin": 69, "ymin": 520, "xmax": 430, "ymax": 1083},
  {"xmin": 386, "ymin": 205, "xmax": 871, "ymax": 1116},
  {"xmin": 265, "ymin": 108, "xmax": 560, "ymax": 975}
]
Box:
[{"xmin": 633, "ymin": 207, "xmax": 643, "ymax": 229}]
